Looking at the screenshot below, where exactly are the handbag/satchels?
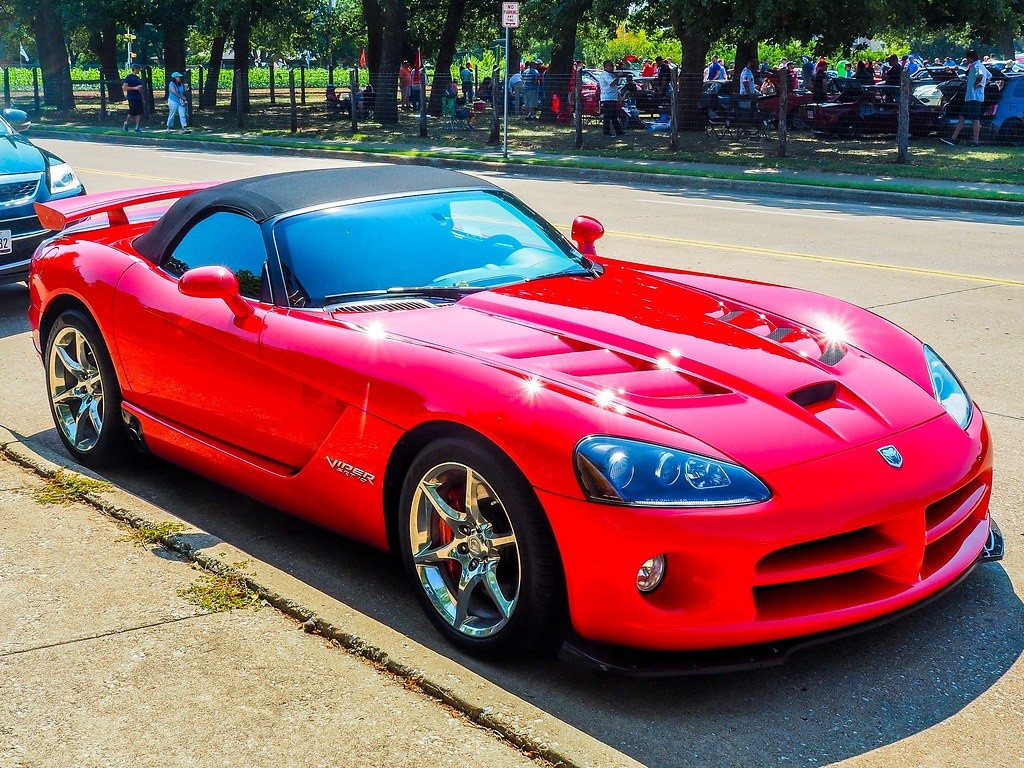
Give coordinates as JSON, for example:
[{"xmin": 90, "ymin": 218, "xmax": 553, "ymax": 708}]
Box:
[
  {"xmin": 656, "ymin": 114, "xmax": 671, "ymax": 123},
  {"xmin": 456, "ymin": 95, "xmax": 466, "ymax": 106},
  {"xmin": 180, "ymin": 97, "xmax": 188, "ymax": 106},
  {"xmin": 626, "ymin": 116, "xmax": 645, "ymax": 129}
]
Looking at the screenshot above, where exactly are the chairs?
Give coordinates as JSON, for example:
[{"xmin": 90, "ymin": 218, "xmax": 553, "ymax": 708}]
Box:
[
  {"xmin": 703, "ymin": 92, "xmax": 772, "ymax": 140},
  {"xmin": 441, "ymin": 97, "xmax": 469, "ymax": 131},
  {"xmin": 583, "ymin": 93, "xmax": 600, "ymax": 129},
  {"xmin": 881, "ymin": 93, "xmax": 894, "ymax": 103},
  {"xmin": 325, "ymin": 99, "xmax": 344, "ymax": 119}
]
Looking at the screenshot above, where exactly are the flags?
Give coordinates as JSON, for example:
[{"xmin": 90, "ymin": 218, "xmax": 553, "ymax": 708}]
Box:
[
  {"xmin": 413, "ymin": 50, "xmax": 424, "ymax": 85},
  {"xmin": 359, "ymin": 48, "xmax": 368, "ymax": 69}
]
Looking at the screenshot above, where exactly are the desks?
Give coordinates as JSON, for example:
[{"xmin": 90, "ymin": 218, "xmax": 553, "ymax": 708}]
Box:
[{"xmin": 472, "ymin": 107, "xmax": 493, "ymax": 132}]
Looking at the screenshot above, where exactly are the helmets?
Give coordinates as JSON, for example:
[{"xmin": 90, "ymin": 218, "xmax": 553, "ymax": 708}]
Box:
[{"xmin": 536, "ymin": 59, "xmax": 543, "ymax": 66}]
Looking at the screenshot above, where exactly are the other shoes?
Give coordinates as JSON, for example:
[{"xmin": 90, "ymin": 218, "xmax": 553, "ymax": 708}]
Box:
[
  {"xmin": 467, "ymin": 125, "xmax": 474, "ymax": 131},
  {"xmin": 123, "ymin": 122, "xmax": 129, "ymax": 132},
  {"xmin": 617, "ymin": 132, "xmax": 628, "ymax": 137},
  {"xmin": 166, "ymin": 131, "xmax": 172, "ymax": 134},
  {"xmin": 528, "ymin": 114, "xmax": 537, "ymax": 121},
  {"xmin": 602, "ymin": 133, "xmax": 616, "ymax": 138},
  {"xmin": 182, "ymin": 130, "xmax": 192, "ymax": 134},
  {"xmin": 134, "ymin": 128, "xmax": 141, "ymax": 134},
  {"xmin": 406, "ymin": 105, "xmax": 413, "ymax": 109},
  {"xmin": 401, "ymin": 106, "xmax": 407, "ymax": 111},
  {"xmin": 413, "ymin": 107, "xmax": 419, "ymax": 111}
]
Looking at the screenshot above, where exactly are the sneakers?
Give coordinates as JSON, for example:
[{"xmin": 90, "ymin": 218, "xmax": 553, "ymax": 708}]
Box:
[
  {"xmin": 963, "ymin": 142, "xmax": 981, "ymax": 150},
  {"xmin": 940, "ymin": 137, "xmax": 956, "ymax": 145}
]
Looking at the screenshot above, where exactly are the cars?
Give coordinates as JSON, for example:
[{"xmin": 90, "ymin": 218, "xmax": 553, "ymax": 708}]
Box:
[
  {"xmin": 0, "ymin": 107, "xmax": 90, "ymax": 283},
  {"xmin": 573, "ymin": 68, "xmax": 626, "ymax": 116},
  {"xmin": 622, "ymin": 71, "xmax": 1024, "ymax": 142}
]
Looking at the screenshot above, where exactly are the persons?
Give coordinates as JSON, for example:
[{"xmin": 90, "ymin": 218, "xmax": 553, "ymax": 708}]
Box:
[
  {"xmin": 477, "ymin": 77, "xmax": 493, "ymax": 108},
  {"xmin": 740, "ymin": 56, "xmax": 759, "ymax": 96},
  {"xmin": 760, "ymin": 72, "xmax": 777, "ymax": 98},
  {"xmin": 703, "ymin": 52, "xmax": 735, "ymax": 82},
  {"xmin": 508, "ymin": 58, "xmax": 547, "ymax": 131},
  {"xmin": 600, "ymin": 60, "xmax": 625, "ymax": 140},
  {"xmin": 845, "ymin": 48, "xmax": 1024, "ymax": 101},
  {"xmin": 165, "ymin": 69, "xmax": 193, "ymax": 133},
  {"xmin": 619, "ymin": 75, "xmax": 637, "ymax": 101},
  {"xmin": 655, "ymin": 57, "xmax": 671, "ymax": 92},
  {"xmin": 640, "ymin": 57, "xmax": 659, "ymax": 89},
  {"xmin": 799, "ymin": 56, "xmax": 815, "ymax": 91},
  {"xmin": 325, "ymin": 84, "xmax": 353, "ymax": 117},
  {"xmin": 399, "ymin": 59, "xmax": 413, "ymax": 109},
  {"xmin": 348, "ymin": 84, "xmax": 364, "ymax": 122},
  {"xmin": 812, "ymin": 54, "xmax": 830, "ymax": 103},
  {"xmin": 760, "ymin": 56, "xmax": 798, "ymax": 93},
  {"xmin": 567, "ymin": 61, "xmax": 582, "ymax": 105},
  {"xmin": 939, "ymin": 49, "xmax": 993, "ymax": 148},
  {"xmin": 442, "ymin": 89, "xmax": 477, "ymax": 130},
  {"xmin": 411, "ymin": 65, "xmax": 421, "ymax": 111},
  {"xmin": 461, "ymin": 63, "xmax": 473, "ymax": 109},
  {"xmin": 452, "ymin": 76, "xmax": 458, "ymax": 97},
  {"xmin": 123, "ymin": 64, "xmax": 143, "ymax": 133}
]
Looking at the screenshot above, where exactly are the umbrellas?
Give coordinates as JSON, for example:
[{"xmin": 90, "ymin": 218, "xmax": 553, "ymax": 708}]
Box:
[{"xmin": 625, "ymin": 54, "xmax": 638, "ymax": 62}]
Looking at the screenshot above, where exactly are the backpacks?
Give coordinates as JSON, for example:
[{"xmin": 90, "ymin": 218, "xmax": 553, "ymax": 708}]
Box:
[{"xmin": 661, "ymin": 65, "xmax": 671, "ymax": 82}]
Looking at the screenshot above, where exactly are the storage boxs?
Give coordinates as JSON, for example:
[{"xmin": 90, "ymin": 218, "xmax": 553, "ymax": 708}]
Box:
[{"xmin": 473, "ymin": 101, "xmax": 485, "ymax": 111}]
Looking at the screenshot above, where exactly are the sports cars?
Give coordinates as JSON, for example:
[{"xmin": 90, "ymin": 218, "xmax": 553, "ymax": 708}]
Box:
[{"xmin": 28, "ymin": 163, "xmax": 1005, "ymax": 677}]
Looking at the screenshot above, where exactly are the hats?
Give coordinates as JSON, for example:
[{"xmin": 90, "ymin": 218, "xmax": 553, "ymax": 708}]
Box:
[
  {"xmin": 132, "ymin": 64, "xmax": 142, "ymax": 68},
  {"xmin": 171, "ymin": 72, "xmax": 183, "ymax": 78},
  {"xmin": 787, "ymin": 61, "xmax": 796, "ymax": 67}
]
